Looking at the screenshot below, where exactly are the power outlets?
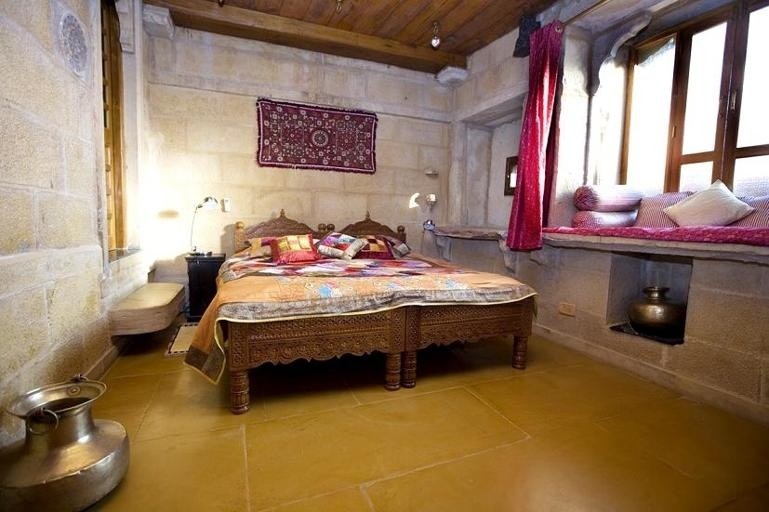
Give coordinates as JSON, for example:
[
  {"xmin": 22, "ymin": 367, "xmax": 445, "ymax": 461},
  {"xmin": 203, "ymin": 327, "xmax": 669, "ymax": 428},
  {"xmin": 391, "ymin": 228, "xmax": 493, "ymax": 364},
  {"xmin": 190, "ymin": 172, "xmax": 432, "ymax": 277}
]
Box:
[{"xmin": 558, "ymin": 302, "xmax": 576, "ymax": 317}]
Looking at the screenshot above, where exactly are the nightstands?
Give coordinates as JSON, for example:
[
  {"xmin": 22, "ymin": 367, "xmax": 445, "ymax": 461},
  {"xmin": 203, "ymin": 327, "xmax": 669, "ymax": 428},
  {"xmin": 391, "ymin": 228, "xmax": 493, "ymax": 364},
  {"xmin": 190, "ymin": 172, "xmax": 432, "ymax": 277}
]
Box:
[{"xmin": 185, "ymin": 253, "xmax": 227, "ymax": 323}]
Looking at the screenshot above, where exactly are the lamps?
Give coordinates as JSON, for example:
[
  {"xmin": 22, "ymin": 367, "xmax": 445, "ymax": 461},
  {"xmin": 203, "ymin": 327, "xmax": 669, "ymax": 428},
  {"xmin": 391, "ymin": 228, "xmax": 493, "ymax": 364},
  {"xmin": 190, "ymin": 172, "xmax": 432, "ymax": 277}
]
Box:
[
  {"xmin": 189, "ymin": 197, "xmax": 231, "ymax": 255},
  {"xmin": 431, "ymin": 20, "xmax": 442, "ymax": 48},
  {"xmin": 425, "ymin": 192, "xmax": 439, "ymax": 210}
]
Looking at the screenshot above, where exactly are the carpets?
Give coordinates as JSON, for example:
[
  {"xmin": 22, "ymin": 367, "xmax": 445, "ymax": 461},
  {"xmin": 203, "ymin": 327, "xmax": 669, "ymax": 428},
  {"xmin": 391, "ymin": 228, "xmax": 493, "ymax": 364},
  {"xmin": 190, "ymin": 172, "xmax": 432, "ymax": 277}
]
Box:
[{"xmin": 164, "ymin": 322, "xmax": 199, "ymax": 357}]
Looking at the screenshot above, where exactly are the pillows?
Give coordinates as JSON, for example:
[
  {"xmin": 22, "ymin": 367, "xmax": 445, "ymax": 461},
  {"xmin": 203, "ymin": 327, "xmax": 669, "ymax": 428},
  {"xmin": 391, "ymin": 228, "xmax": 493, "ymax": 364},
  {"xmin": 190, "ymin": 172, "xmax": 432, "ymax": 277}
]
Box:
[
  {"xmin": 352, "ymin": 234, "xmax": 396, "ymax": 260},
  {"xmin": 270, "ymin": 233, "xmax": 321, "ymax": 265},
  {"xmin": 314, "ymin": 230, "xmax": 368, "ymax": 260},
  {"xmin": 382, "ymin": 234, "xmax": 412, "ymax": 257},
  {"xmin": 246, "ymin": 236, "xmax": 276, "ymax": 259},
  {"xmin": 571, "ymin": 180, "xmax": 757, "ymax": 227}
]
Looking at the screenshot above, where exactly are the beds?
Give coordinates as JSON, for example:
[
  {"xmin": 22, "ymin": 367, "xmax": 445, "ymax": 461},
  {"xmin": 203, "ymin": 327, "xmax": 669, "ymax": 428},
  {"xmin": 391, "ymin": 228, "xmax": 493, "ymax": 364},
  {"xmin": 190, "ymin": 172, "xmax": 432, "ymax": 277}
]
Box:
[
  {"xmin": 225, "ymin": 207, "xmax": 406, "ymax": 413},
  {"xmin": 327, "ymin": 211, "xmax": 538, "ymax": 388}
]
